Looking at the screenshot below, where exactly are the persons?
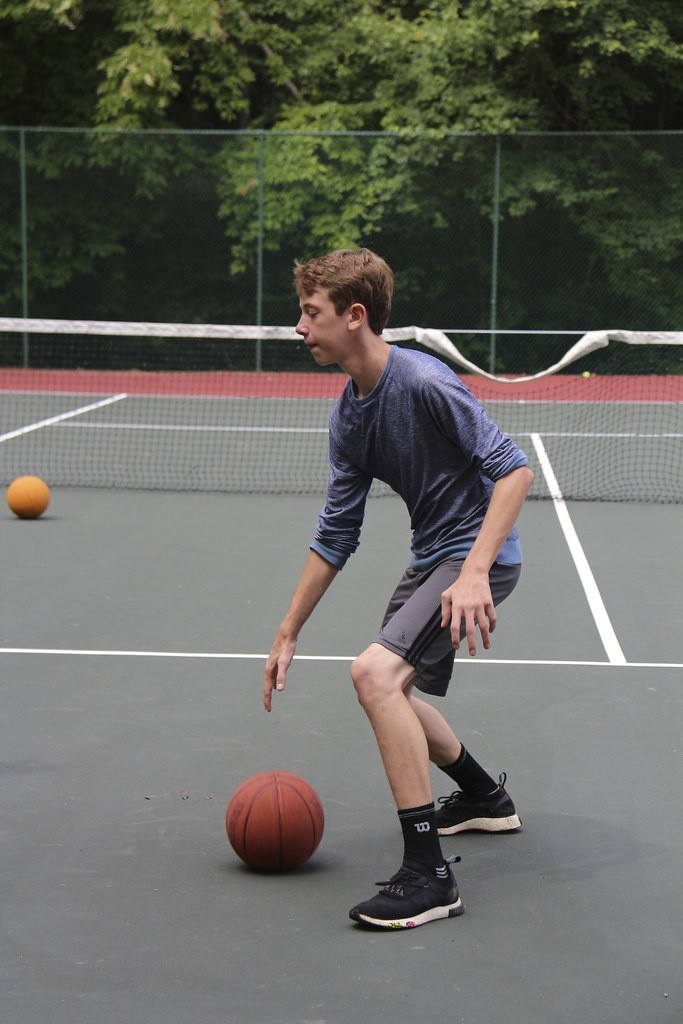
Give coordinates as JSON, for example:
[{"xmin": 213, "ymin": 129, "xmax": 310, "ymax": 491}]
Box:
[{"xmin": 263, "ymin": 248, "xmax": 534, "ymax": 930}]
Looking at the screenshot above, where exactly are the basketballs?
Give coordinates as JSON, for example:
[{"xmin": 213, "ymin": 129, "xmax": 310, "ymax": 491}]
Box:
[
  {"xmin": 224, "ymin": 770, "xmax": 326, "ymax": 871},
  {"xmin": 7, "ymin": 476, "xmax": 51, "ymax": 518}
]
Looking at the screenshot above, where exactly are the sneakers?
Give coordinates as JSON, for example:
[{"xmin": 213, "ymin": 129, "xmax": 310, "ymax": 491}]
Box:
[
  {"xmin": 348, "ymin": 856, "xmax": 463, "ymax": 929},
  {"xmin": 433, "ymin": 771, "xmax": 521, "ymax": 836}
]
